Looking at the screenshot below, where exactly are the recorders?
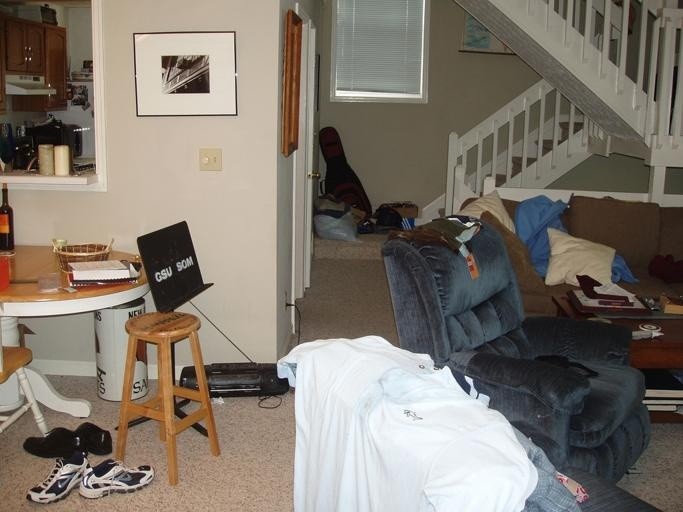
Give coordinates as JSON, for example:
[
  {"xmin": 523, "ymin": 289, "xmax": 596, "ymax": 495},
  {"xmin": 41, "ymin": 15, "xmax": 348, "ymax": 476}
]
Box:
[{"xmin": 179, "ymin": 362, "xmax": 290, "ymax": 398}]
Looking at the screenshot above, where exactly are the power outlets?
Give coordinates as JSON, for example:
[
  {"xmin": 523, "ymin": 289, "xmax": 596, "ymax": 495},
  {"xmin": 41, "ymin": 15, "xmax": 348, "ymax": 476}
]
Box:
[{"xmin": 281, "ymin": 289, "xmax": 288, "ymax": 313}]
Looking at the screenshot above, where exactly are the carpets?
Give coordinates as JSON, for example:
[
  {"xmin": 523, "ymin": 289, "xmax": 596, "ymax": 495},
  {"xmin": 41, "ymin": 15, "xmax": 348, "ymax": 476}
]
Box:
[{"xmin": 313, "ymin": 232, "xmax": 392, "ymax": 262}]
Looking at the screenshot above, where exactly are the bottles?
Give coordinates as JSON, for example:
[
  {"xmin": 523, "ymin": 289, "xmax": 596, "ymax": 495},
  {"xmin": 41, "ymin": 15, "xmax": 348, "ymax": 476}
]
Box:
[
  {"xmin": 13, "ymin": 124, "xmax": 71, "ymax": 176},
  {"xmin": 0, "ymin": 182, "xmax": 15, "ymax": 251}
]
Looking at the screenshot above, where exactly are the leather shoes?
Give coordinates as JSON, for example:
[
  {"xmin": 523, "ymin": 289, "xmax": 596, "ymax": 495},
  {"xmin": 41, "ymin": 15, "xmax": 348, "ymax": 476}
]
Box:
[
  {"xmin": 75, "ymin": 421, "xmax": 115, "ymax": 456},
  {"xmin": 22, "ymin": 425, "xmax": 88, "ymax": 465}
]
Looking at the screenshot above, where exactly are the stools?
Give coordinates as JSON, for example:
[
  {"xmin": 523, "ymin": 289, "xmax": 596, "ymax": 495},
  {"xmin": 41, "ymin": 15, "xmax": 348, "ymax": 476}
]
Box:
[
  {"xmin": 0, "ymin": 345, "xmax": 50, "ymax": 438},
  {"xmin": 116, "ymin": 311, "xmax": 222, "ymax": 488}
]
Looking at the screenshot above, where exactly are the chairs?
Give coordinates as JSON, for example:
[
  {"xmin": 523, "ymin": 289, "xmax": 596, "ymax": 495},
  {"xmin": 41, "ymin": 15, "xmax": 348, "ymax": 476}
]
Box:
[
  {"xmin": 284, "ymin": 338, "xmax": 663, "ymax": 512},
  {"xmin": 380, "ymin": 214, "xmax": 653, "ymax": 491}
]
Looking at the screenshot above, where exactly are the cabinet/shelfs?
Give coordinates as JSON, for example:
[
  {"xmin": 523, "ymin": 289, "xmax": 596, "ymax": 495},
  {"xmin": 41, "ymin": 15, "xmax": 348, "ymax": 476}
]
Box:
[{"xmin": 0, "ymin": 12, "xmax": 68, "ymax": 115}]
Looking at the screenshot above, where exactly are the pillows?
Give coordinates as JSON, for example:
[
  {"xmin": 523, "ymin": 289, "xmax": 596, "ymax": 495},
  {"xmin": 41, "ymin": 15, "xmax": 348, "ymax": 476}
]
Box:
[{"xmin": 459, "ymin": 189, "xmax": 683, "ymax": 296}]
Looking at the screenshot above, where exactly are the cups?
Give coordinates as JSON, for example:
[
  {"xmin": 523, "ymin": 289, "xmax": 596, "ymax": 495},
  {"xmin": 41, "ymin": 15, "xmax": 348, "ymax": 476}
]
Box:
[{"xmin": 38, "ymin": 272, "xmax": 59, "ymax": 293}]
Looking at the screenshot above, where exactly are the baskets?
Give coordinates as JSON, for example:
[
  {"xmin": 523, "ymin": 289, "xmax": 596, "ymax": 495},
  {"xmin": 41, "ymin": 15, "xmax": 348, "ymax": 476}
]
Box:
[{"xmin": 51, "ymin": 233, "xmax": 117, "ymax": 274}]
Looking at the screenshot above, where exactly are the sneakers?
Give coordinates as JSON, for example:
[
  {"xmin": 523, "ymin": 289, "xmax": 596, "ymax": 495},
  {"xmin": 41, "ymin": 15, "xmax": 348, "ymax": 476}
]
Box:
[
  {"xmin": 79, "ymin": 458, "xmax": 156, "ymax": 500},
  {"xmin": 26, "ymin": 450, "xmax": 91, "ymax": 505}
]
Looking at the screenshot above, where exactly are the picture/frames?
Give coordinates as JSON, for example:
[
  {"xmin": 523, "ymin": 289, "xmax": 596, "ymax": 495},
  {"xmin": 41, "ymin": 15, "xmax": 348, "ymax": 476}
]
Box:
[
  {"xmin": 133, "ymin": 30, "xmax": 237, "ymax": 117},
  {"xmin": 280, "ymin": 8, "xmax": 303, "ymax": 158}
]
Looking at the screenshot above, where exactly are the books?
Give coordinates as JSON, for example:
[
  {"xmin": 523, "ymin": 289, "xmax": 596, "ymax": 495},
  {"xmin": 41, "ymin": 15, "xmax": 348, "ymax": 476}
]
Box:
[
  {"xmin": 567, "ymin": 285, "xmax": 652, "ymax": 315},
  {"xmin": 638, "ymin": 369, "xmax": 683, "ymax": 412},
  {"xmin": 65, "ymin": 259, "xmax": 140, "ymax": 288},
  {"xmin": 660, "ymin": 295, "xmax": 683, "ymax": 314}
]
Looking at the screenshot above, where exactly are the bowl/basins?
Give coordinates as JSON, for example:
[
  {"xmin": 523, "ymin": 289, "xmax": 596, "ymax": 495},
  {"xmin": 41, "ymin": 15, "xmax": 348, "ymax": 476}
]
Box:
[{"xmin": 70, "ymin": 72, "xmax": 94, "ymax": 81}]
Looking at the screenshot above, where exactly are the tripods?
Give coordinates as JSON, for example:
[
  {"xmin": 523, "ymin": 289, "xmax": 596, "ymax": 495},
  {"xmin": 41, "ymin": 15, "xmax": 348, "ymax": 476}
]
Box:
[{"xmin": 115, "ymin": 342, "xmax": 208, "ymax": 437}]
